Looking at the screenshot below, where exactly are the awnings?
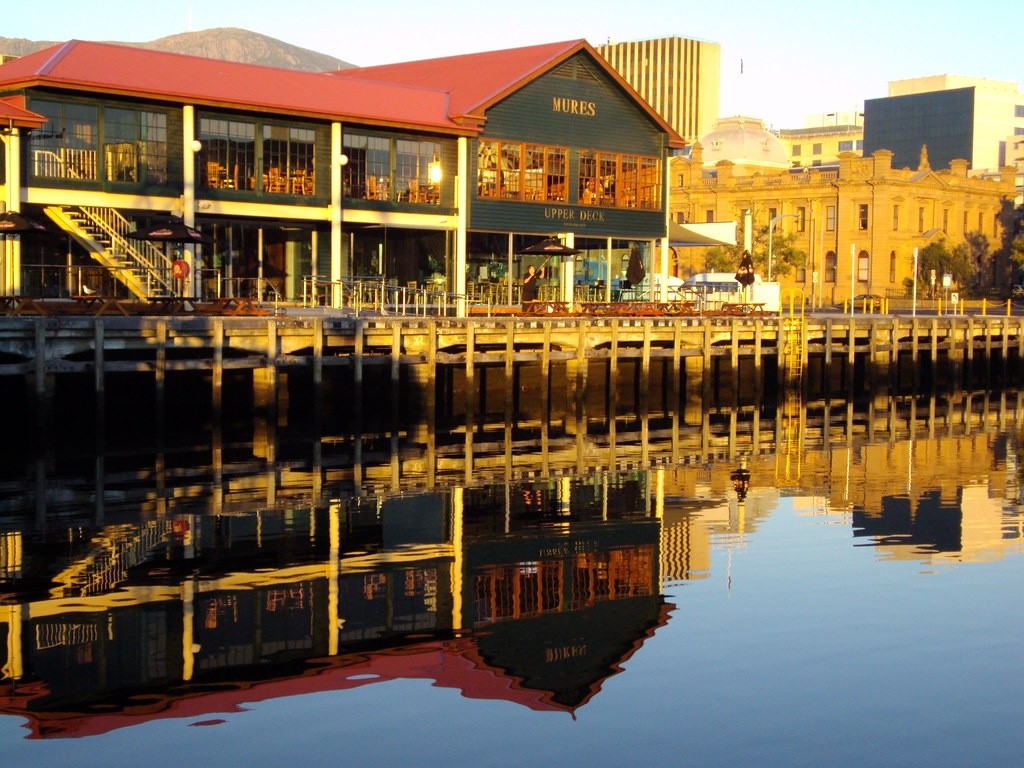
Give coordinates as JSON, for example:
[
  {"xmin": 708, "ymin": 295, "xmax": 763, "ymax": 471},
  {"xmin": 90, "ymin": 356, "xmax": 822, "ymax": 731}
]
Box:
[{"xmin": 657, "ymin": 219, "xmax": 736, "ymax": 246}]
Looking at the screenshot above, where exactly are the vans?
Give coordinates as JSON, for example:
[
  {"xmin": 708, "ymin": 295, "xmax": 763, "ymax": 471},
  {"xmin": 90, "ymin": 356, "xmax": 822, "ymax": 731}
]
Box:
[{"xmin": 675, "ymin": 272, "xmax": 766, "ymax": 310}]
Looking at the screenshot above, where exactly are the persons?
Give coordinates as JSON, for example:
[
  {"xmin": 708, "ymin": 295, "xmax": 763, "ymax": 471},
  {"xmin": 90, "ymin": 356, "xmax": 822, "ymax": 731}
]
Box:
[{"xmin": 523, "ymin": 266, "xmax": 545, "ymax": 312}]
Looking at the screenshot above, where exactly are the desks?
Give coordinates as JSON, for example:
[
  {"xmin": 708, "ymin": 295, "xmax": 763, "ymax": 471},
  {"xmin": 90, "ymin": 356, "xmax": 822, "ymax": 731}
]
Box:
[
  {"xmin": 147, "ymin": 297, "xmax": 200, "ymax": 317},
  {"xmin": 0, "ymin": 295, "xmax": 45, "ymax": 316},
  {"xmin": 523, "ymin": 300, "xmax": 766, "ymax": 315},
  {"xmin": 208, "ymin": 298, "xmax": 262, "ymax": 317},
  {"xmin": 71, "ymin": 295, "xmax": 129, "ymax": 316}
]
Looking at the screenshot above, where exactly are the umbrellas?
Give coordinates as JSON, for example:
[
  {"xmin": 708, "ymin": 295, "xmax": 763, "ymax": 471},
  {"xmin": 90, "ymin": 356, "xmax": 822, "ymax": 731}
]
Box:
[
  {"xmin": 735, "ymin": 250, "xmax": 755, "ymax": 313},
  {"xmin": 626, "ymin": 246, "xmax": 645, "ymax": 285},
  {"xmin": 0, "ymin": 212, "xmax": 51, "ymax": 295},
  {"xmin": 123, "ymin": 222, "xmax": 217, "ymax": 261},
  {"xmin": 521, "ymin": 240, "xmax": 583, "ymax": 287}
]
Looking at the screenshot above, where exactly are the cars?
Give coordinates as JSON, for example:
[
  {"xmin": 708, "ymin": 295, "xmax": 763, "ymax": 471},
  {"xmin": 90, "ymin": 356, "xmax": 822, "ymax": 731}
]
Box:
[
  {"xmin": 840, "ymin": 294, "xmax": 884, "ymax": 310},
  {"xmin": 1013, "ymin": 285, "xmax": 1024, "ymax": 298}
]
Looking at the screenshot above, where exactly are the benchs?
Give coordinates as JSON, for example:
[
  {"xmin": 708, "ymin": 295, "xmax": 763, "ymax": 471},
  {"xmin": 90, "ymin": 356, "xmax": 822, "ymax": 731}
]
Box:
[
  {"xmin": 515, "ymin": 311, "xmax": 781, "ymax": 317},
  {"xmin": 0, "ymin": 302, "xmax": 272, "ymax": 316}
]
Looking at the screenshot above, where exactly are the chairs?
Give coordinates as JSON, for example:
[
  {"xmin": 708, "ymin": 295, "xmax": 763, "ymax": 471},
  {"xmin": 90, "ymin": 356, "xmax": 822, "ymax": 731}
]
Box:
[
  {"xmin": 479, "ymin": 178, "xmax": 650, "ymax": 208},
  {"xmin": 297, "ymin": 277, "xmax": 634, "ymax": 304},
  {"xmin": 204, "ymin": 567, "xmax": 424, "ymax": 628},
  {"xmin": 201, "ymin": 161, "xmax": 440, "ymax": 206}
]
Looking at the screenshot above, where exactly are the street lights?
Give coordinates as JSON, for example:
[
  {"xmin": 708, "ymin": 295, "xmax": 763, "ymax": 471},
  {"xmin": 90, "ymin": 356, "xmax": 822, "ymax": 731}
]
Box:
[{"xmin": 768, "ymin": 213, "xmax": 801, "ymax": 308}]
